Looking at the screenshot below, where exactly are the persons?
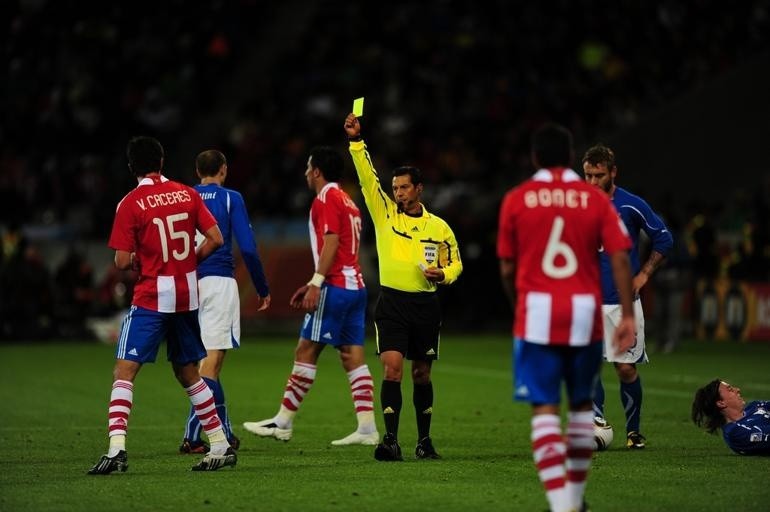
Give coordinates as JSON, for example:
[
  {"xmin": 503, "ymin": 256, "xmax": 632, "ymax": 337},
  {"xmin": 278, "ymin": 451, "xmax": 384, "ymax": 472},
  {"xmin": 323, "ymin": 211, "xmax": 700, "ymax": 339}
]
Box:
[
  {"xmin": 0, "ymin": 106, "xmax": 769, "ymax": 348},
  {"xmin": 177, "ymin": 149, "xmax": 272, "ymax": 454},
  {"xmin": 342, "ymin": 112, "xmax": 465, "ymax": 461},
  {"xmin": 495, "ymin": 123, "xmax": 636, "ymax": 511},
  {"xmin": 238, "ymin": 144, "xmax": 385, "ymax": 447},
  {"xmin": 581, "ymin": 143, "xmax": 676, "ymax": 448},
  {"xmin": 87, "ymin": 134, "xmax": 240, "ymax": 476},
  {"xmin": 691, "ymin": 377, "xmax": 770, "ymax": 456}
]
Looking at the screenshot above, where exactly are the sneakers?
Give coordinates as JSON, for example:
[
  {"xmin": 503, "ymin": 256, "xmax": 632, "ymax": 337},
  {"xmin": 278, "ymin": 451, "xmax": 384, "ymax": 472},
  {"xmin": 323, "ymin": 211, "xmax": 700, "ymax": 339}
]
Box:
[
  {"xmin": 179, "ymin": 435, "xmax": 239, "ymax": 471},
  {"xmin": 331, "ymin": 431, "xmax": 379, "ymax": 446},
  {"xmin": 375, "ymin": 433, "xmax": 401, "ymax": 461},
  {"xmin": 88, "ymin": 450, "xmax": 128, "ymax": 475},
  {"xmin": 243, "ymin": 418, "xmax": 293, "ymax": 441},
  {"xmin": 415, "ymin": 436, "xmax": 440, "ymax": 459},
  {"xmin": 627, "ymin": 431, "xmax": 646, "ymax": 448}
]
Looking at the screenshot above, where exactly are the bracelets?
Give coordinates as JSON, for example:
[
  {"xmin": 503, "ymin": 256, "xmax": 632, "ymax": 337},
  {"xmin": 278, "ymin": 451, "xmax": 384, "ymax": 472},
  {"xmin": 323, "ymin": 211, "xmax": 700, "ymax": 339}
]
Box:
[{"xmin": 309, "ymin": 272, "xmax": 325, "ymax": 288}]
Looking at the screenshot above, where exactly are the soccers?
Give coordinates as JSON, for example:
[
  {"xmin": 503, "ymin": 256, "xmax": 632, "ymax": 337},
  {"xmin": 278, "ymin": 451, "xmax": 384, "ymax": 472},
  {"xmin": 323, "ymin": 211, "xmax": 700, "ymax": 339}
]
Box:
[{"xmin": 593, "ymin": 415, "xmax": 613, "ymax": 451}]
